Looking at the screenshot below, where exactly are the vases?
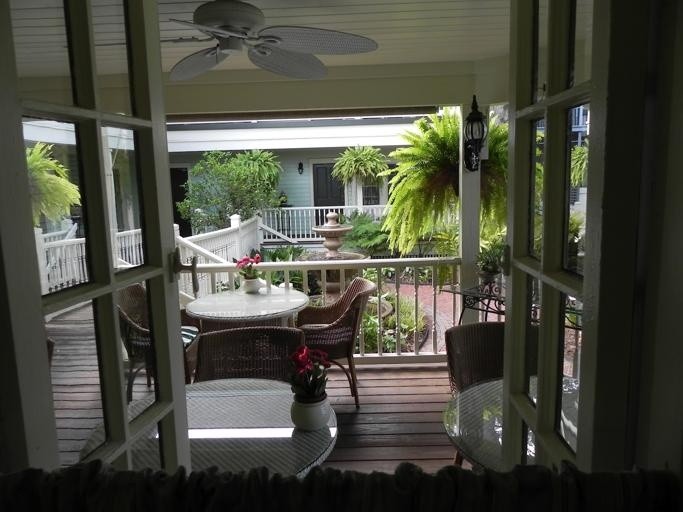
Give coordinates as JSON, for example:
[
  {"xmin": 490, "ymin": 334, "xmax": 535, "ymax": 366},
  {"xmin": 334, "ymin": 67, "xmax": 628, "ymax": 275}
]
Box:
[
  {"xmin": 242, "ymin": 278, "xmax": 262, "ymax": 293},
  {"xmin": 291, "ymin": 393, "xmax": 330, "ymax": 431}
]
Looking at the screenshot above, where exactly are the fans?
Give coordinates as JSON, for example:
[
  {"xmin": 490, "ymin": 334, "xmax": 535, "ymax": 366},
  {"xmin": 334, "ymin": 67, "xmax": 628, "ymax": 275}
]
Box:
[{"xmin": 63, "ymin": 0, "xmax": 378, "ymax": 82}]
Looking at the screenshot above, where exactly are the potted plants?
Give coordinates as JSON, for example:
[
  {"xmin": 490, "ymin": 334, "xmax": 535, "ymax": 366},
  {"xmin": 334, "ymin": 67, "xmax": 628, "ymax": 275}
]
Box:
[{"xmin": 477, "ymin": 235, "xmax": 505, "ymax": 273}]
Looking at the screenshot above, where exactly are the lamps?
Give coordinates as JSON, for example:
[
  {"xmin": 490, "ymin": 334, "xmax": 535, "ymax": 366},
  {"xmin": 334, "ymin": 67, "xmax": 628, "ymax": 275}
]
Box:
[
  {"xmin": 464, "ymin": 95, "xmax": 488, "ymax": 171},
  {"xmin": 298, "ymin": 162, "xmax": 304, "ymax": 174}
]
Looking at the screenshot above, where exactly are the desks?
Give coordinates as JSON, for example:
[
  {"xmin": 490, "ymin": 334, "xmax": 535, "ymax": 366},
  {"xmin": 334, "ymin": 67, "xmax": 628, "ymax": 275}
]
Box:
[
  {"xmin": 438, "ymin": 270, "xmax": 582, "ymax": 348},
  {"xmin": 445, "ymin": 375, "xmax": 581, "ymax": 474}
]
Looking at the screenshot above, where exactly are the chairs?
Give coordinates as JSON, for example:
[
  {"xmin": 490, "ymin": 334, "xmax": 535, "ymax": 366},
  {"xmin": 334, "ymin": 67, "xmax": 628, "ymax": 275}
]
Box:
[
  {"xmin": 111, "ymin": 284, "xmax": 203, "ymax": 406},
  {"xmin": 297, "ymin": 277, "xmax": 377, "ymax": 408},
  {"xmin": 192, "ymin": 326, "xmax": 305, "ymax": 383},
  {"xmin": 445, "ymin": 322, "xmax": 539, "ymax": 467}
]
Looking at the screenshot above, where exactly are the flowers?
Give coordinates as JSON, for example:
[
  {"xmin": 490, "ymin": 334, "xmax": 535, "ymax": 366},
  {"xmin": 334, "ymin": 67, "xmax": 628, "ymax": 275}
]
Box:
[
  {"xmin": 283, "ymin": 345, "xmax": 331, "ymax": 398},
  {"xmin": 237, "ymin": 254, "xmax": 261, "ymax": 279}
]
